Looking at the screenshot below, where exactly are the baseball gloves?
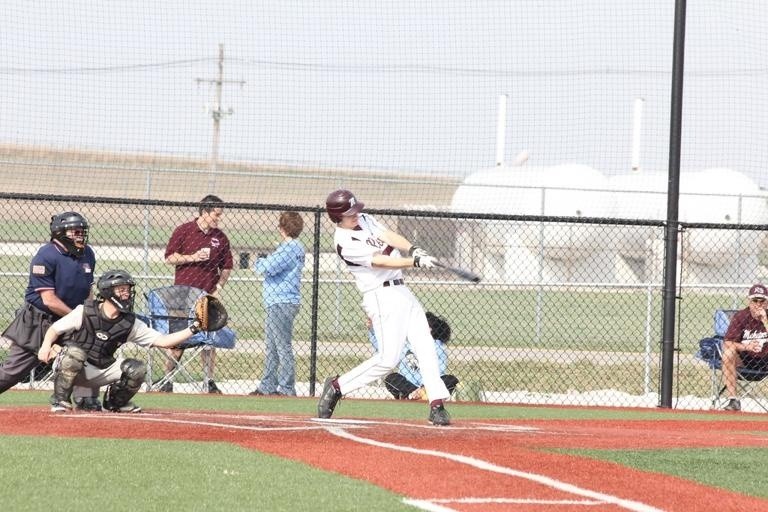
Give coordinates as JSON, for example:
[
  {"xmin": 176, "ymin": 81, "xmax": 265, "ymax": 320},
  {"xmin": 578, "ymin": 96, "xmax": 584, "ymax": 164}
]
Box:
[{"xmin": 195, "ymin": 295, "xmax": 227, "ymax": 331}]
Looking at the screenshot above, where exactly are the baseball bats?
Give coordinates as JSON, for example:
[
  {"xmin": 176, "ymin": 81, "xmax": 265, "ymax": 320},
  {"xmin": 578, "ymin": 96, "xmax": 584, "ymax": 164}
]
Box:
[{"xmin": 431, "ymin": 261, "xmax": 481, "ymax": 283}]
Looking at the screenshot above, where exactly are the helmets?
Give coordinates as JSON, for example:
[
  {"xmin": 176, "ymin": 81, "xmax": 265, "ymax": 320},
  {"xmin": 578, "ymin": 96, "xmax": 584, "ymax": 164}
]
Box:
[
  {"xmin": 326, "ymin": 189, "xmax": 365, "ymax": 218},
  {"xmin": 96, "ymin": 269, "xmax": 137, "ymax": 313},
  {"xmin": 50, "ymin": 212, "xmax": 89, "ymax": 258}
]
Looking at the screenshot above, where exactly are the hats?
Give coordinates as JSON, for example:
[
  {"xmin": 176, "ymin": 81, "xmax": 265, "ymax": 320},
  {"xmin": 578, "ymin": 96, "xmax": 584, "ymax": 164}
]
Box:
[{"xmin": 748, "ymin": 285, "xmax": 768, "ymax": 301}]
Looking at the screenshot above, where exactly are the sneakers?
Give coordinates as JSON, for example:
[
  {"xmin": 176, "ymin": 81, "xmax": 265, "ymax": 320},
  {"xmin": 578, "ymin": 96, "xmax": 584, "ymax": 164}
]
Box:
[
  {"xmin": 249, "ymin": 389, "xmax": 297, "ymax": 396},
  {"xmin": 317, "ymin": 375, "xmax": 341, "ymax": 419},
  {"xmin": 723, "ymin": 398, "xmax": 741, "ymax": 411},
  {"xmin": 202, "ymin": 381, "xmax": 221, "ymax": 395},
  {"xmin": 428, "ymin": 404, "xmax": 450, "ymax": 427},
  {"xmin": 52, "ymin": 397, "xmax": 142, "ymax": 414},
  {"xmin": 147, "ymin": 379, "xmax": 173, "ymax": 392}
]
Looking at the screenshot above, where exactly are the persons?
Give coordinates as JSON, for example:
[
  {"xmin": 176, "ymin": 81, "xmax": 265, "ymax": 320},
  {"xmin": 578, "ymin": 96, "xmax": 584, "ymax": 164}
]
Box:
[
  {"xmin": 0, "ymin": 211, "xmax": 103, "ymax": 413},
  {"xmin": 154, "ymin": 193, "xmax": 233, "ymax": 395},
  {"xmin": 720, "ymin": 283, "xmax": 767, "ymax": 414},
  {"xmin": 245, "ymin": 211, "xmax": 307, "ymax": 398},
  {"xmin": 366, "ymin": 311, "xmax": 460, "ymax": 402},
  {"xmin": 317, "ymin": 190, "xmax": 454, "ymax": 428},
  {"xmin": 36, "ymin": 269, "xmax": 229, "ymax": 414}
]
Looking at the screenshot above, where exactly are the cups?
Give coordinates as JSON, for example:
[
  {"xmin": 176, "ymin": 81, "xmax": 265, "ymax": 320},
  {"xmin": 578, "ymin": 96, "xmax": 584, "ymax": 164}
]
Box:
[
  {"xmin": 755, "ymin": 339, "xmax": 764, "ymax": 352},
  {"xmin": 200, "ymin": 248, "xmax": 211, "ymax": 260}
]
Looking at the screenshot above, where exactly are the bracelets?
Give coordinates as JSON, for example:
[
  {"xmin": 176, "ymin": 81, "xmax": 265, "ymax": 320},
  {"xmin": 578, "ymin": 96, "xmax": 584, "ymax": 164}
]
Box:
[{"xmin": 215, "ymin": 283, "xmax": 223, "ymax": 290}]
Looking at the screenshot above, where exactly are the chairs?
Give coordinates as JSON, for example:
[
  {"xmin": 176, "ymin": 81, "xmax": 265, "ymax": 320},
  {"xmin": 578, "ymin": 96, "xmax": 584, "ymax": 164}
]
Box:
[
  {"xmin": 711, "ymin": 308, "xmax": 768, "ymax": 412},
  {"xmin": 146, "ymin": 286, "xmax": 236, "ymax": 393}
]
